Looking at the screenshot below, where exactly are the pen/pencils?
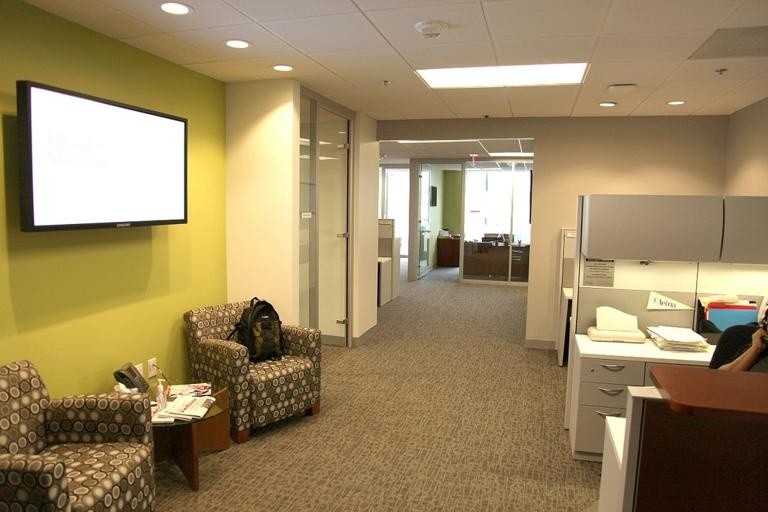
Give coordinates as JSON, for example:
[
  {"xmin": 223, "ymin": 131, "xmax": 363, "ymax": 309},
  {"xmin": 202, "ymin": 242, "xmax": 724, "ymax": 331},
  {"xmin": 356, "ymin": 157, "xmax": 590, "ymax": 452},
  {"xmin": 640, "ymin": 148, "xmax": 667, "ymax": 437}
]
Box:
[{"xmin": 211, "ymin": 388, "xmax": 228, "ymax": 397}]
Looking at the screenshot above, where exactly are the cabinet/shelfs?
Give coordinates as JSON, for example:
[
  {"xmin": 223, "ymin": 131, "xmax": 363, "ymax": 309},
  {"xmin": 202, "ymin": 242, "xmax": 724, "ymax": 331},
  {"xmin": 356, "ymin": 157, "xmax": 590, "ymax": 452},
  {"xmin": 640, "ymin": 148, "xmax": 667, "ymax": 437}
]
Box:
[{"xmin": 436, "ymin": 234, "xmax": 460, "ymax": 267}]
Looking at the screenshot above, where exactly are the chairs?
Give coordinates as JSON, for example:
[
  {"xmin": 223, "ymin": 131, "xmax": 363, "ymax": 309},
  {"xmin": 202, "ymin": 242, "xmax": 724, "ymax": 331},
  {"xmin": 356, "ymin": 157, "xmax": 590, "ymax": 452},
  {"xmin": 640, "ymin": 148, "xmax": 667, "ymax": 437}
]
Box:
[
  {"xmin": 0, "ymin": 357, "xmax": 158, "ymax": 512},
  {"xmin": 181, "ymin": 300, "xmax": 324, "ymax": 445}
]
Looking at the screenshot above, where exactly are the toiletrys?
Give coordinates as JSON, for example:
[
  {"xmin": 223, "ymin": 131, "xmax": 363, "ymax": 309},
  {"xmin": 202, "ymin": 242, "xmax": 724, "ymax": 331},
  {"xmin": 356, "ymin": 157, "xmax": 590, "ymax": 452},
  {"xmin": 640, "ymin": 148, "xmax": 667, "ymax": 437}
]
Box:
[{"xmin": 155, "ymin": 379, "xmax": 166, "ymax": 413}]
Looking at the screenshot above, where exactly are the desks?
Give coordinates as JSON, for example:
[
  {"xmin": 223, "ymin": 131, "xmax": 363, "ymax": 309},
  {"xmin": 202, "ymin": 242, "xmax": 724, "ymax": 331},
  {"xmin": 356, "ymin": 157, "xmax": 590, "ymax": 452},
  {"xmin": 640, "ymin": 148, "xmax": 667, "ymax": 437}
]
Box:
[
  {"xmin": 463, "ymin": 242, "xmax": 531, "ymax": 280},
  {"xmin": 151, "ymin": 389, "xmax": 232, "ymax": 492},
  {"xmin": 568, "ymin": 333, "xmax": 722, "ymax": 464}
]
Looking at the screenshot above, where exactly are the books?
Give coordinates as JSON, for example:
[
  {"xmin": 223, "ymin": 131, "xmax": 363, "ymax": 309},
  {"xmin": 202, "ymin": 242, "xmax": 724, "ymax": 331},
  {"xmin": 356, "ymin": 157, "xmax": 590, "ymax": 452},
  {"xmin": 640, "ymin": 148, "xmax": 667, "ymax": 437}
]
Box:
[
  {"xmin": 149, "ymin": 402, "xmax": 175, "ymax": 424},
  {"xmin": 164, "ymin": 381, "xmax": 211, "ymax": 397},
  {"xmin": 644, "ymin": 325, "xmax": 708, "ymax": 353}
]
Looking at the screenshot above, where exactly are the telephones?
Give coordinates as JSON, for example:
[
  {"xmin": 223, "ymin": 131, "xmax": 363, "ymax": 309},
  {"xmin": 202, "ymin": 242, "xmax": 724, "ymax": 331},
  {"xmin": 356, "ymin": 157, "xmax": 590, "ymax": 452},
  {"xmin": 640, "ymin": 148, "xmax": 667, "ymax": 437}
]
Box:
[
  {"xmin": 114, "ymin": 362, "xmax": 150, "ymax": 394},
  {"xmin": 761, "ymin": 324, "xmax": 768, "ymax": 344}
]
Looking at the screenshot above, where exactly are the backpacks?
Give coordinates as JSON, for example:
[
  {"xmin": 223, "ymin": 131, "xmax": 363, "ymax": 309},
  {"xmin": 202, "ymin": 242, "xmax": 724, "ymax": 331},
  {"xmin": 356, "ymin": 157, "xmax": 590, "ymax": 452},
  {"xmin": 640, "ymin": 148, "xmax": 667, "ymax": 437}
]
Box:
[{"xmin": 237, "ymin": 297, "xmax": 285, "ymax": 363}]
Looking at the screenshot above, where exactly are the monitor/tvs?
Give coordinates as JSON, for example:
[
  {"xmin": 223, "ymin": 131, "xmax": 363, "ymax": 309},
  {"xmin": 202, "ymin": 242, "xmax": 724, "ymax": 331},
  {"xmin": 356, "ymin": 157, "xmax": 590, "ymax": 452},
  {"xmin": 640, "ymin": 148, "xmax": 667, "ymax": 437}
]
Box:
[{"xmin": 16, "ymin": 80, "xmax": 187, "ymax": 232}]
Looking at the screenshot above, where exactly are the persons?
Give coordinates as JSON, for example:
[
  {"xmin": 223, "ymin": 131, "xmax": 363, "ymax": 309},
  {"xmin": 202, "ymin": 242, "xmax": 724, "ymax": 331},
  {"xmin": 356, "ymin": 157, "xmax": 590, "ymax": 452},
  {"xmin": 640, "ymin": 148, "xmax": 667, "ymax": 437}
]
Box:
[{"xmin": 708, "ymin": 306, "xmax": 767, "ymax": 373}]
